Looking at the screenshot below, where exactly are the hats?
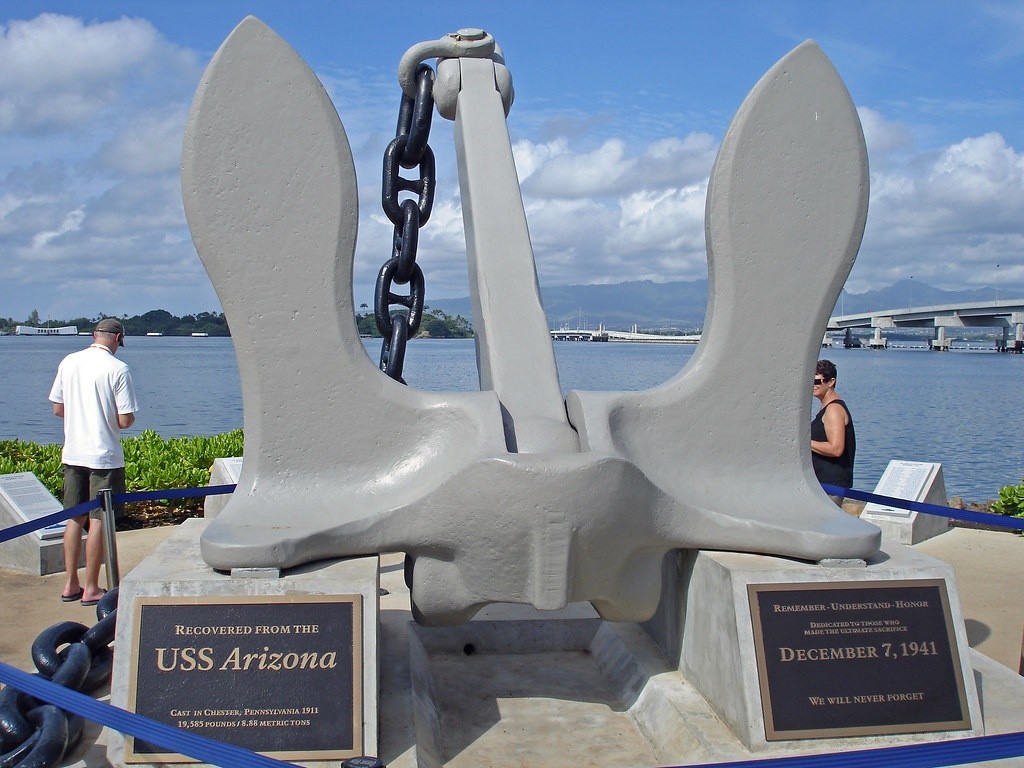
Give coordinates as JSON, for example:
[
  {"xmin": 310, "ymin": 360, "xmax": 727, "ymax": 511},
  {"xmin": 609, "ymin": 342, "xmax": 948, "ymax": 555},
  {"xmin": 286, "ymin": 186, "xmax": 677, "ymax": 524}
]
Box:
[{"xmin": 95, "ymin": 319, "xmax": 125, "ymax": 348}]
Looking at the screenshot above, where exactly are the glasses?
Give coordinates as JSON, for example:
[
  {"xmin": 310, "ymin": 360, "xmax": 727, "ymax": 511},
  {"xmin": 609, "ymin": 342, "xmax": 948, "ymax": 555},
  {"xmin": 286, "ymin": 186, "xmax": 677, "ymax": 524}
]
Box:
[{"xmin": 813, "ymin": 379, "xmax": 831, "ymax": 385}]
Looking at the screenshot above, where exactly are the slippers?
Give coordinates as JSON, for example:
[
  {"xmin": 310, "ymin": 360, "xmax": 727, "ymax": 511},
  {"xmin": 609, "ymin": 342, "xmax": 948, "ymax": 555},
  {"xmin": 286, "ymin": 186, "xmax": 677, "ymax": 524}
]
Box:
[
  {"xmin": 81, "ymin": 589, "xmax": 108, "ymax": 606},
  {"xmin": 62, "ymin": 587, "xmax": 84, "ymax": 602}
]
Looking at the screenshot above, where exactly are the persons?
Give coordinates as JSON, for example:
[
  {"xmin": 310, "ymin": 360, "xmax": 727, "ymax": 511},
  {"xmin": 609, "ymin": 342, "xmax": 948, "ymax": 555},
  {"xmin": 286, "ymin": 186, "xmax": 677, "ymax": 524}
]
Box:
[
  {"xmin": 811, "ymin": 360, "xmax": 856, "ymax": 507},
  {"xmin": 49, "ymin": 318, "xmax": 138, "ymax": 606}
]
